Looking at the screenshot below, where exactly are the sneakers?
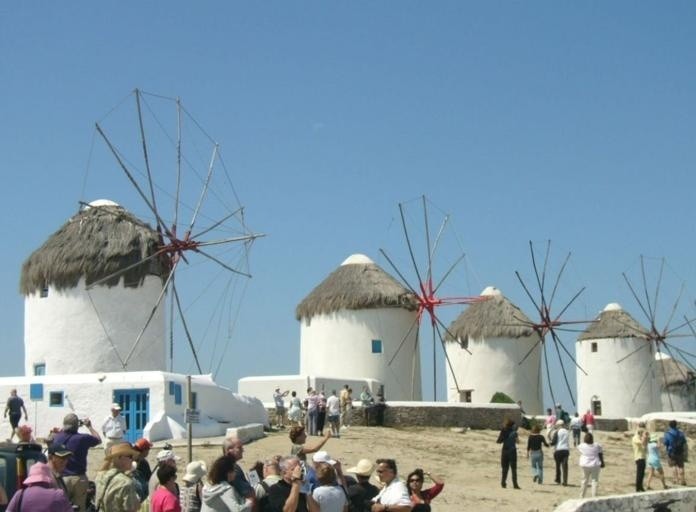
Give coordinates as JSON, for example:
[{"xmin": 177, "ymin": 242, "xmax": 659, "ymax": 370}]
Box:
[
  {"xmin": 636, "ymin": 480, "xmax": 688, "ymax": 492},
  {"xmin": 501, "ymin": 475, "xmax": 569, "ymax": 489}
]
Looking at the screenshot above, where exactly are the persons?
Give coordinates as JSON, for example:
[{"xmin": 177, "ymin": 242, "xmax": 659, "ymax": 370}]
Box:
[
  {"xmin": 526, "ymin": 404, "xmax": 669, "ymax": 498},
  {"xmin": 663, "ymin": 420, "xmax": 688, "ymax": 486},
  {"xmin": 496, "ymin": 418, "xmax": 521, "ymax": 489}
]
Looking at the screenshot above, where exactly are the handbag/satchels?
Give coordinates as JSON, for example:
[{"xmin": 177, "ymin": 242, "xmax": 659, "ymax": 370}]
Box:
[{"xmin": 599, "ymin": 452, "xmax": 606, "ymax": 467}]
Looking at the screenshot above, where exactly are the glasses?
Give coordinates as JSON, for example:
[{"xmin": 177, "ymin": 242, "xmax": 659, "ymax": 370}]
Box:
[
  {"xmin": 409, "ymin": 478, "xmax": 423, "ymax": 482},
  {"xmin": 124, "ymin": 454, "xmax": 133, "ymax": 460}
]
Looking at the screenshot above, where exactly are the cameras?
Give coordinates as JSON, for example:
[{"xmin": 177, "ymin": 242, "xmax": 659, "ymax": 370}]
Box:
[
  {"xmin": 78, "ymin": 421, "xmax": 85, "ymax": 425},
  {"xmin": 69, "ymin": 502, "xmax": 80, "ymax": 512}
]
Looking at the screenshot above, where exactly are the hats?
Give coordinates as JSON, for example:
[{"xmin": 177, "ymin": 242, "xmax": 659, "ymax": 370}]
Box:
[
  {"xmin": 136, "ymin": 438, "xmax": 153, "ymax": 451},
  {"xmin": 155, "ymin": 449, "xmax": 182, "ymax": 463},
  {"xmin": 110, "ymin": 402, "xmax": 122, "ymax": 410},
  {"xmin": 345, "ymin": 458, "xmax": 377, "ymax": 478},
  {"xmin": 104, "ymin": 442, "xmax": 141, "ymax": 461},
  {"xmin": 312, "ymin": 450, "xmax": 337, "ymax": 466},
  {"xmin": 48, "ymin": 443, "xmax": 72, "ymax": 457},
  {"xmin": 181, "ymin": 460, "xmax": 207, "ymax": 485},
  {"xmin": 22, "ymin": 462, "xmax": 57, "ymax": 485}
]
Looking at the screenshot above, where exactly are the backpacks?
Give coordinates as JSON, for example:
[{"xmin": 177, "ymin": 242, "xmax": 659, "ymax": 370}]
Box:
[{"xmin": 666, "ymin": 429, "xmax": 686, "ymax": 461}]
